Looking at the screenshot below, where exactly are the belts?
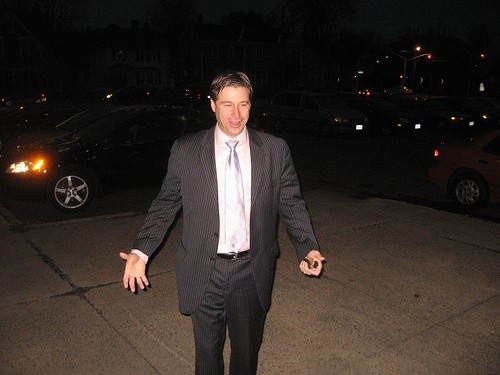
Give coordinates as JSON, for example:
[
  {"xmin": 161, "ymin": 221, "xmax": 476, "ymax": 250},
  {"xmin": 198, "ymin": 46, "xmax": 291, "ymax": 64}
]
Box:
[{"xmin": 215, "ymin": 250, "xmax": 249, "ymax": 260}]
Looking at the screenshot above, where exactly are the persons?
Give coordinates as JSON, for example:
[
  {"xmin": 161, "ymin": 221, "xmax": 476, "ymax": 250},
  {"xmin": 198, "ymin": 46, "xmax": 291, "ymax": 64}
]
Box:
[{"xmin": 119, "ymin": 72, "xmax": 324, "ymax": 375}]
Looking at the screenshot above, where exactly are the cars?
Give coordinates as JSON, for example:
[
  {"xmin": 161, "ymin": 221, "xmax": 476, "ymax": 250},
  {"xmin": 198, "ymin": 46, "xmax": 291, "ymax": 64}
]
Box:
[
  {"xmin": 421, "ymin": 128, "xmax": 500, "ymax": 212},
  {"xmin": 386, "ymin": 86, "xmax": 414, "ymax": 95},
  {"xmin": 417, "ymin": 97, "xmax": 473, "ymax": 134},
  {"xmin": 339, "ymin": 91, "xmax": 425, "ymax": 138},
  {"xmin": 163, "ymin": 83, "xmax": 211, "ymax": 108},
  {"xmin": 0, "ymin": 104, "xmax": 265, "ymax": 213},
  {"xmin": 469, "ymin": 95, "xmax": 500, "ymax": 133},
  {"xmin": 264, "ymin": 89, "xmax": 370, "ymax": 139}
]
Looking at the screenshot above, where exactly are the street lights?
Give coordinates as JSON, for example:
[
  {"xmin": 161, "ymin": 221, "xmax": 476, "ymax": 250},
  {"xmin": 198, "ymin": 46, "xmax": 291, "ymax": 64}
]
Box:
[{"xmin": 385, "ymin": 48, "xmax": 433, "ymax": 85}]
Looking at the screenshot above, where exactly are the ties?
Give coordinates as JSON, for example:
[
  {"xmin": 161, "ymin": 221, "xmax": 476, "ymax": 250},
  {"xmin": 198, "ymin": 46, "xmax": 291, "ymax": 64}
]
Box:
[{"xmin": 222, "ymin": 139, "xmax": 246, "ymax": 251}]
